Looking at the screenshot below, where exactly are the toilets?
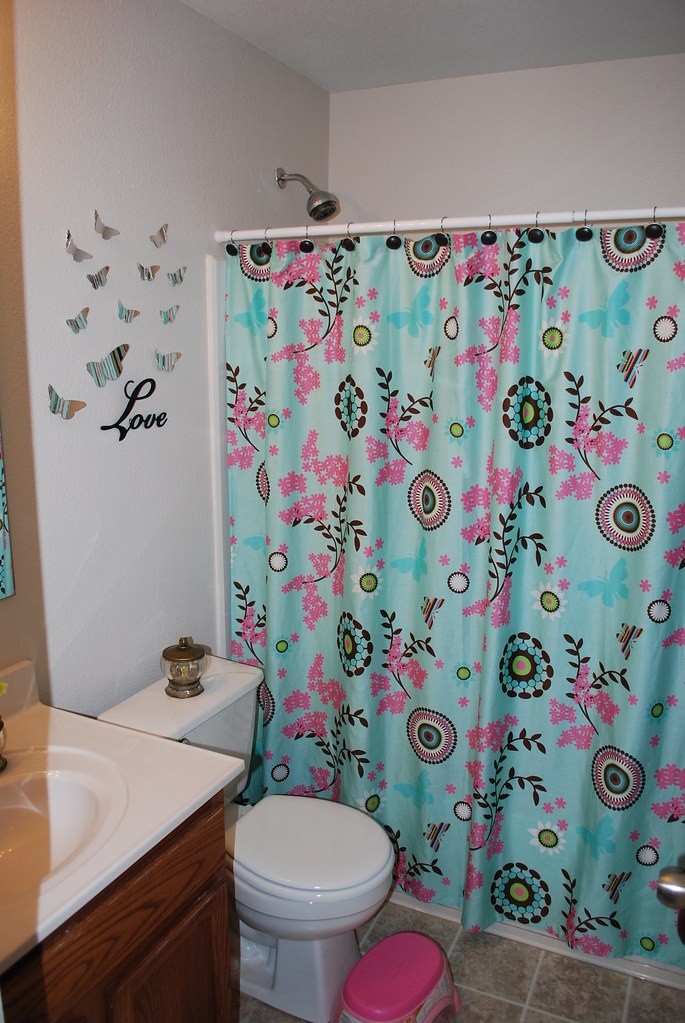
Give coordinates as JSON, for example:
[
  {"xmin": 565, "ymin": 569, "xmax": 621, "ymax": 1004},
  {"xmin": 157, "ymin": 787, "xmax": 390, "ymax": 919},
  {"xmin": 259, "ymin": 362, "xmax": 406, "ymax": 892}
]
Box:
[{"xmin": 95, "ymin": 651, "xmax": 396, "ymax": 1023}]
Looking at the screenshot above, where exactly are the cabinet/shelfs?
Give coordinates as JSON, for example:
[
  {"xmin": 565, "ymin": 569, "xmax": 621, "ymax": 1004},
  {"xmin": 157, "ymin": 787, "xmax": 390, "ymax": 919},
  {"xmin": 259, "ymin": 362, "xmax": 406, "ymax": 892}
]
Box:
[{"xmin": 0, "ymin": 786, "xmax": 234, "ymax": 1023}]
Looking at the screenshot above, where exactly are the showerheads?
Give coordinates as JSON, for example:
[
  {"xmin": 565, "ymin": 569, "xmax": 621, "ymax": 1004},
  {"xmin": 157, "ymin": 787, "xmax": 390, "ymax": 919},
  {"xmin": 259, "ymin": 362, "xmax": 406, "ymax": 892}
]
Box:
[{"xmin": 277, "ymin": 167, "xmax": 342, "ymax": 223}]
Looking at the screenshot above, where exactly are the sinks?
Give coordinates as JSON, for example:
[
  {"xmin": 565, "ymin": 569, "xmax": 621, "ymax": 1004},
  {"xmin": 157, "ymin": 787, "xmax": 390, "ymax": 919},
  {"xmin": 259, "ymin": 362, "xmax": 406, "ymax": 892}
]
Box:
[{"xmin": 0, "ymin": 745, "xmax": 130, "ymax": 907}]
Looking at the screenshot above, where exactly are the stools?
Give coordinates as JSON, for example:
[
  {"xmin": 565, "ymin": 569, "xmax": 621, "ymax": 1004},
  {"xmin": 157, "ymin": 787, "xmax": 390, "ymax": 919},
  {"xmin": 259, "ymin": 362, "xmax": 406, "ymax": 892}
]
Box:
[{"xmin": 330, "ymin": 931, "xmax": 461, "ymax": 1022}]
